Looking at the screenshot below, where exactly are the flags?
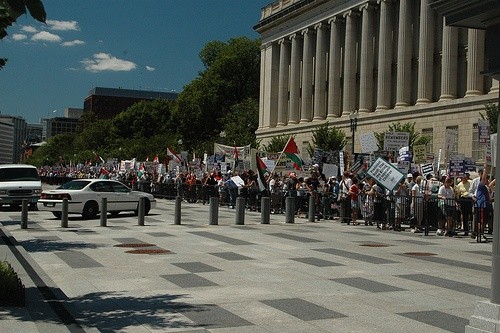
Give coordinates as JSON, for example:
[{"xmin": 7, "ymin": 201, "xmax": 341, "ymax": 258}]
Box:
[
  {"xmin": 138, "ymin": 165, "xmax": 145, "ymax": 177},
  {"xmin": 234, "ymin": 148, "xmax": 239, "ymax": 169},
  {"xmin": 284, "ymin": 137, "xmax": 304, "ymax": 172},
  {"xmin": 256, "ymin": 154, "xmax": 268, "ymax": 192}
]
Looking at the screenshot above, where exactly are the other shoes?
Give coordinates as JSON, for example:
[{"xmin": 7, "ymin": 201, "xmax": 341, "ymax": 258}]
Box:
[
  {"xmin": 394, "ymin": 226, "xmax": 401, "ymax": 231},
  {"xmin": 475, "ymin": 235, "xmax": 488, "ymax": 242},
  {"xmin": 399, "ymin": 225, "xmax": 405, "ymax": 231}
]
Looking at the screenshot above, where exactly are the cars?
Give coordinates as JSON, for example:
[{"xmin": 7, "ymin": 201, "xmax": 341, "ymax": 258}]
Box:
[{"xmin": 37, "ymin": 179, "xmax": 157, "ymax": 219}]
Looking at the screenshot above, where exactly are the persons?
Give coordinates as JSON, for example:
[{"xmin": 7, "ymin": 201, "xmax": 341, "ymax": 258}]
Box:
[{"xmin": 39, "ymin": 164, "xmax": 495, "ymax": 243}]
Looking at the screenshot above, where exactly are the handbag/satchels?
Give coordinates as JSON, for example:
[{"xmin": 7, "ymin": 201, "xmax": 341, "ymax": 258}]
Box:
[{"xmin": 410, "ymin": 217, "xmax": 417, "ymax": 228}]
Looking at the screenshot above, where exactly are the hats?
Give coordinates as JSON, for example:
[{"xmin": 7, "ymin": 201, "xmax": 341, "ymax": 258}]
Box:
[
  {"xmin": 291, "ymin": 173, "xmax": 297, "ymax": 177},
  {"xmin": 407, "ymin": 174, "xmax": 413, "ymax": 177},
  {"xmin": 442, "ymin": 173, "xmax": 447, "ymax": 176},
  {"xmin": 312, "ymin": 164, "xmax": 319, "ymax": 168},
  {"xmin": 331, "ymin": 176, "xmax": 337, "ymax": 180}
]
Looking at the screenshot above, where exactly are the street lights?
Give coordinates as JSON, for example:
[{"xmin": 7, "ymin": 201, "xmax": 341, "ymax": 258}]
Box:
[
  {"xmin": 119, "ymin": 147, "xmax": 123, "ymax": 162},
  {"xmin": 349, "ymin": 109, "xmax": 359, "ymax": 155},
  {"xmin": 219, "ymin": 130, "xmax": 226, "ymax": 145},
  {"xmin": 177, "ymin": 138, "xmax": 182, "ymax": 151},
  {"xmin": 74, "ymin": 153, "xmax": 77, "ymax": 168}
]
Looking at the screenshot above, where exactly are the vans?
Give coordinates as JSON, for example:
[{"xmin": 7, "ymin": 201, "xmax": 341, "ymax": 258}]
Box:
[{"xmin": 0, "ymin": 164, "xmax": 42, "ymax": 210}]
